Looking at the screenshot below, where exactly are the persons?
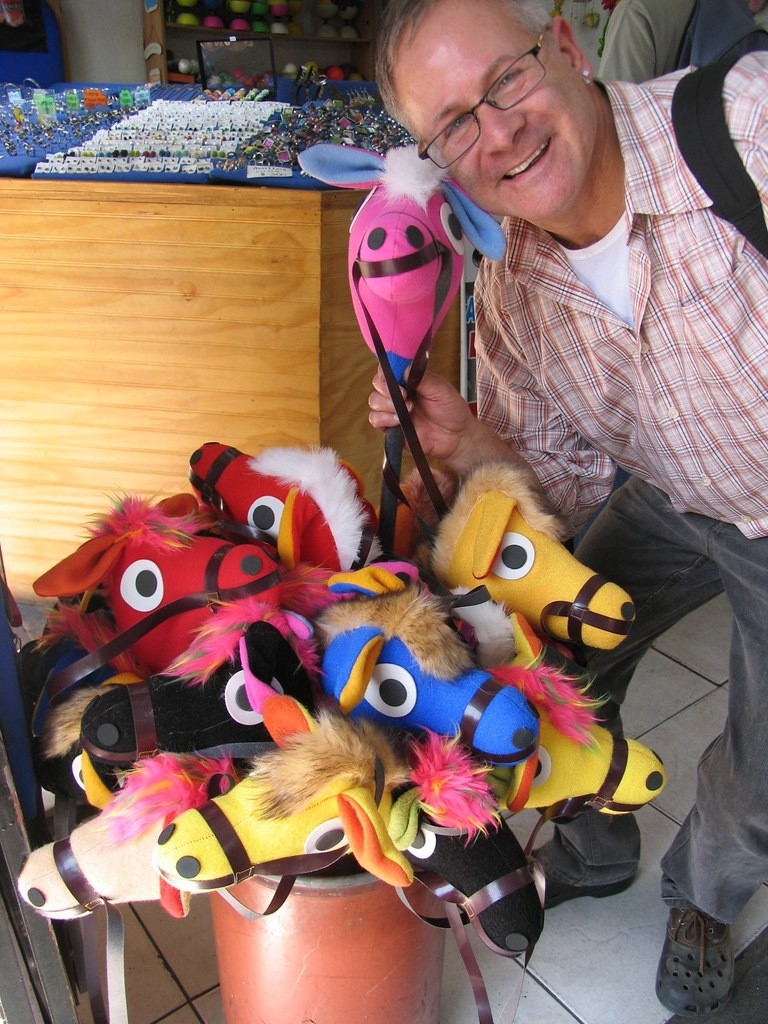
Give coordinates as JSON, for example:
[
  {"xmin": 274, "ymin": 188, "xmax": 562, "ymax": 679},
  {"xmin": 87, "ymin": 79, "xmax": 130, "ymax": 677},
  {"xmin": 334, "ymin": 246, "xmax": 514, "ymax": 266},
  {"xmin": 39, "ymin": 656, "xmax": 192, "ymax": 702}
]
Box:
[
  {"xmin": 598, "ymin": 0, "xmax": 768, "ymax": 85},
  {"xmin": 368, "ymin": 0, "xmax": 768, "ymax": 1019}
]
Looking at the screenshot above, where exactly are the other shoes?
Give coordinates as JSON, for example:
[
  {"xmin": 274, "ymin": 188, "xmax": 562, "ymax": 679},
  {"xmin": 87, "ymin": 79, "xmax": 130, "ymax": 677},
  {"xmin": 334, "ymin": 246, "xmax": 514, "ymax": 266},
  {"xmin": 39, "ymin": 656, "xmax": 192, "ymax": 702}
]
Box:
[
  {"xmin": 529, "ymin": 848, "xmax": 635, "ymax": 909},
  {"xmin": 655, "ymin": 908, "xmax": 735, "ymax": 1020}
]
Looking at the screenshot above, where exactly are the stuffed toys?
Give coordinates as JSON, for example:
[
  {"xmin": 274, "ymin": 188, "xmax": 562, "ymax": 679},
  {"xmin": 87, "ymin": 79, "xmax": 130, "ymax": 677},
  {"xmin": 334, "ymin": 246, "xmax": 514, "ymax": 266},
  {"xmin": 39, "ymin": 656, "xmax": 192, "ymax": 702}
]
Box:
[
  {"xmin": 297, "ymin": 142, "xmax": 507, "ymax": 389},
  {"xmin": 18, "ymin": 441, "xmax": 665, "ymax": 958}
]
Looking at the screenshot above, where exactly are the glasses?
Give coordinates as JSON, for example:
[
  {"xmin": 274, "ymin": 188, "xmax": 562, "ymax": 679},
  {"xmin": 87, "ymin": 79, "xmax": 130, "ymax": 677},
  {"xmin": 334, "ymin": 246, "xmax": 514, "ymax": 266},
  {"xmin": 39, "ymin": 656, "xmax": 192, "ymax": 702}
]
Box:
[{"xmin": 418, "ymin": 25, "xmax": 551, "ymax": 169}]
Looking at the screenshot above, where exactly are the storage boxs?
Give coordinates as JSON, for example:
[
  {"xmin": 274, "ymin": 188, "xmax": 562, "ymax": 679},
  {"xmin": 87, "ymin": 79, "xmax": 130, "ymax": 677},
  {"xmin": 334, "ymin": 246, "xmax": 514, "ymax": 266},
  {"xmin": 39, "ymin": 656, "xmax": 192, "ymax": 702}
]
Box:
[{"xmin": 195, "ymin": 36, "xmax": 279, "ymax": 100}]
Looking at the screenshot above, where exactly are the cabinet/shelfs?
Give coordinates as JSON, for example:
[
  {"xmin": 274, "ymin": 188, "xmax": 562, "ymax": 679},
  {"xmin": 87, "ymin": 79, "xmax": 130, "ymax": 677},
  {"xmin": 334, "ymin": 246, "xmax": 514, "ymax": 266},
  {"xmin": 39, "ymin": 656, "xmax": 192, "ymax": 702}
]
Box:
[{"xmin": 142, "ymin": 1, "xmax": 385, "ymax": 89}]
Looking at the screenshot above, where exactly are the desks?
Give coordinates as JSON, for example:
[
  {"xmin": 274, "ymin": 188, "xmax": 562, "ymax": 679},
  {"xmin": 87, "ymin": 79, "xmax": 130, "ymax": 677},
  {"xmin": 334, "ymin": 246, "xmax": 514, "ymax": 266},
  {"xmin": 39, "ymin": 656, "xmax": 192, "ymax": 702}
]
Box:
[{"xmin": 1, "ymin": 173, "xmax": 466, "ymax": 600}]
